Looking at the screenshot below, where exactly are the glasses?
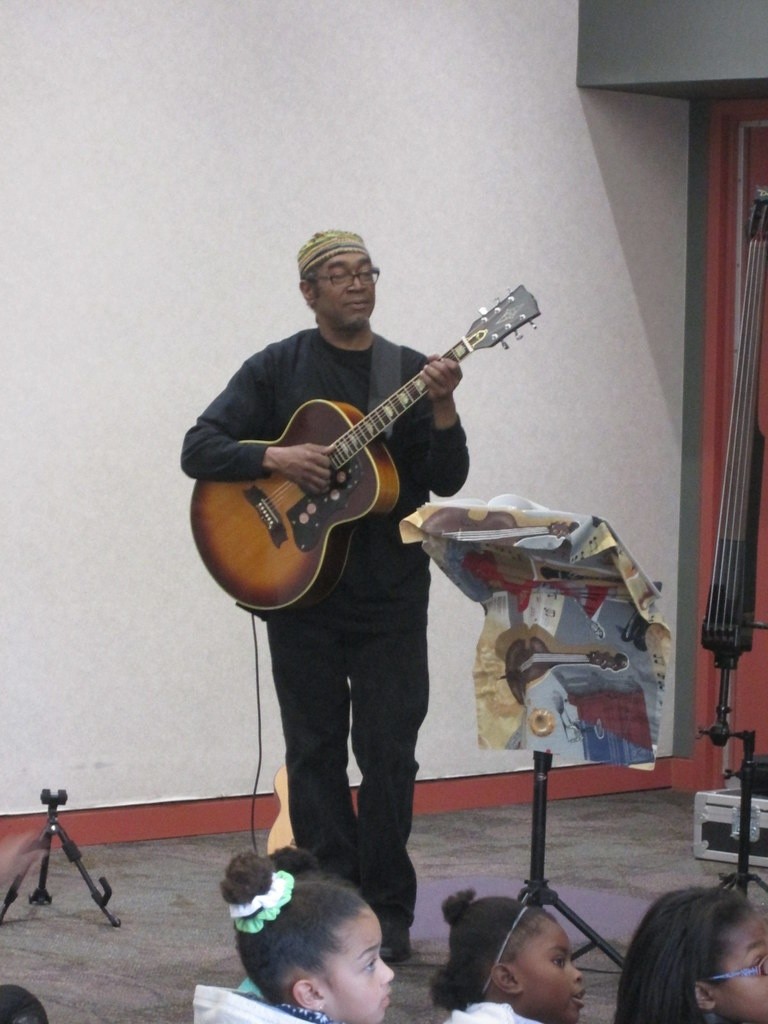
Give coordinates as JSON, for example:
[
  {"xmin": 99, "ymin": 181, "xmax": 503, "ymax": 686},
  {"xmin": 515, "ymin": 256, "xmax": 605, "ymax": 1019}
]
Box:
[
  {"xmin": 311, "ymin": 267, "xmax": 379, "ymax": 288},
  {"xmin": 707, "ymin": 956, "xmax": 768, "ymax": 982}
]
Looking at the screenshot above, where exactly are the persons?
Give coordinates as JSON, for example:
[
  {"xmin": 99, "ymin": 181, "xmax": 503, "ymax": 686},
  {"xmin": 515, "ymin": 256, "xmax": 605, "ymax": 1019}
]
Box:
[
  {"xmin": 431, "ymin": 888, "xmax": 586, "ymax": 1024},
  {"xmin": 614, "ymin": 887, "xmax": 768, "ymax": 1024},
  {"xmin": 193, "ymin": 852, "xmax": 394, "ymax": 1024},
  {"xmin": 181, "ymin": 229, "xmax": 471, "ymax": 958}
]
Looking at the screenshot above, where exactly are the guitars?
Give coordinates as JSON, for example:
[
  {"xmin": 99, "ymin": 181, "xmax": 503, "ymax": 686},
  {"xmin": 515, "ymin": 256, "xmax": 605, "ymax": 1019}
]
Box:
[{"xmin": 186, "ymin": 281, "xmax": 543, "ymax": 624}]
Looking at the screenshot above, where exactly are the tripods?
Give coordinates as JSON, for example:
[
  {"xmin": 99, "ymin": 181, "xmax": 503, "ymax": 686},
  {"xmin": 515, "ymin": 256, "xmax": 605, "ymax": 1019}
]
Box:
[
  {"xmin": 420, "ymin": 502, "xmax": 671, "ymax": 976},
  {"xmin": 715, "ymin": 729, "xmax": 768, "ymax": 907},
  {"xmin": 0, "ymin": 788, "xmax": 120, "ymax": 927}
]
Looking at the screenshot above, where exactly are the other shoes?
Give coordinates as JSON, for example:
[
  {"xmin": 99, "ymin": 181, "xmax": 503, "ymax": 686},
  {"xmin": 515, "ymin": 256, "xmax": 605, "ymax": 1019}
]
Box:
[{"xmin": 382, "ymin": 937, "xmax": 412, "ymax": 959}]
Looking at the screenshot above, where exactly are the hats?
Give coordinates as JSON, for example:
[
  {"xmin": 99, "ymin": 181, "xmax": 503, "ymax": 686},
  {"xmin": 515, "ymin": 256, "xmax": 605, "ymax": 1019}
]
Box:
[{"xmin": 296, "ymin": 229, "xmax": 369, "ymax": 279}]
